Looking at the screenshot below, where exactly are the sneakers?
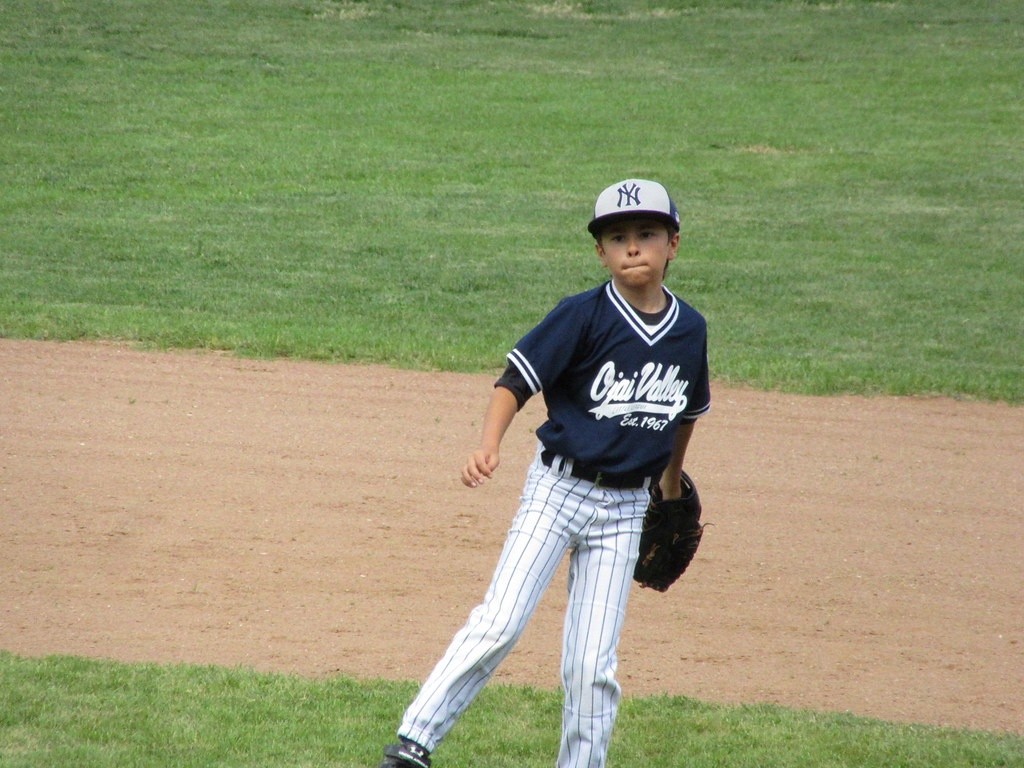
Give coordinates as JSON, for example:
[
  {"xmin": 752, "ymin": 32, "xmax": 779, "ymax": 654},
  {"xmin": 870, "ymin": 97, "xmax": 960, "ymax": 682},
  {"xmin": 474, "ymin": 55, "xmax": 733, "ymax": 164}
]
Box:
[{"xmin": 382, "ymin": 739, "xmax": 432, "ymax": 768}]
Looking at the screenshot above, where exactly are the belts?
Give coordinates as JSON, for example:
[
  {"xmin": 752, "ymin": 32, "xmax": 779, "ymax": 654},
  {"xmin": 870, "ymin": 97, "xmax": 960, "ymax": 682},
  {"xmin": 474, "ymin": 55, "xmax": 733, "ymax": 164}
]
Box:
[{"xmin": 540, "ymin": 450, "xmax": 645, "ymax": 491}]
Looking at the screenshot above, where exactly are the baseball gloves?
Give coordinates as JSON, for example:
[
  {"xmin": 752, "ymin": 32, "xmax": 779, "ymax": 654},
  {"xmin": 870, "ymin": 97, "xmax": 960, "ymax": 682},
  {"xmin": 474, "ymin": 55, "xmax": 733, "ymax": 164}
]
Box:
[{"xmin": 632, "ymin": 463, "xmax": 704, "ymax": 592}]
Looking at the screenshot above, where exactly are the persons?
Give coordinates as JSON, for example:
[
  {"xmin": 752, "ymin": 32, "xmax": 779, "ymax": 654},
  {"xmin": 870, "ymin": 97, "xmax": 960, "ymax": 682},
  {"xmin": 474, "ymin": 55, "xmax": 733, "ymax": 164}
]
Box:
[{"xmin": 383, "ymin": 178, "xmax": 711, "ymax": 768}]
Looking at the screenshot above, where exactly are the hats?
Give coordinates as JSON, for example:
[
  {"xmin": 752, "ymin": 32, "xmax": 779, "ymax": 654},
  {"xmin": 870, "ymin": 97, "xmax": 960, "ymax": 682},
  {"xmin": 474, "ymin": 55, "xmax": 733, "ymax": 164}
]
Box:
[{"xmin": 588, "ymin": 177, "xmax": 680, "ymax": 231}]
